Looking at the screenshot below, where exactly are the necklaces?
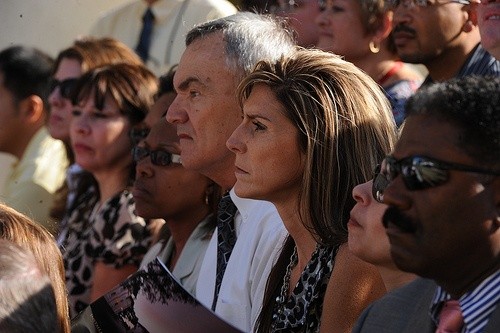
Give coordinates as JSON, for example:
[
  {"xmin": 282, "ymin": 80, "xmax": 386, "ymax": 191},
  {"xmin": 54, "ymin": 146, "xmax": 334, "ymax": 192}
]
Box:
[{"xmin": 377, "ymin": 62, "xmax": 402, "ymax": 85}]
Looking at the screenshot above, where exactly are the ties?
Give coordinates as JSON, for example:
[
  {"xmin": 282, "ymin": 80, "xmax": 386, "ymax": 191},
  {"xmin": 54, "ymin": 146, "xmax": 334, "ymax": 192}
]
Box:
[
  {"xmin": 136, "ymin": 9, "xmax": 154, "ymax": 62},
  {"xmin": 436, "ymin": 301, "xmax": 464, "ymax": 333},
  {"xmin": 211, "ymin": 193, "xmax": 237, "ymax": 312}
]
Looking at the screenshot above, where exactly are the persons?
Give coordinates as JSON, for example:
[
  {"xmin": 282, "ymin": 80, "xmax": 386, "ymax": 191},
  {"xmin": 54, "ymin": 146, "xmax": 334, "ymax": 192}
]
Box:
[{"xmin": 0, "ymin": 0, "xmax": 500, "ymax": 333}]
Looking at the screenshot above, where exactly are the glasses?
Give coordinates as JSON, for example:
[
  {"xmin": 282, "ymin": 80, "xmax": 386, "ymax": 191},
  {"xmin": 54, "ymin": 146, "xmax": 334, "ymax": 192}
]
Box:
[
  {"xmin": 134, "ymin": 147, "xmax": 183, "ymax": 166},
  {"xmin": 43, "ymin": 77, "xmax": 78, "ymax": 99},
  {"xmin": 372, "ymin": 155, "xmax": 500, "ymax": 203},
  {"xmin": 384, "ymin": 0, "xmax": 470, "ymax": 10}
]
